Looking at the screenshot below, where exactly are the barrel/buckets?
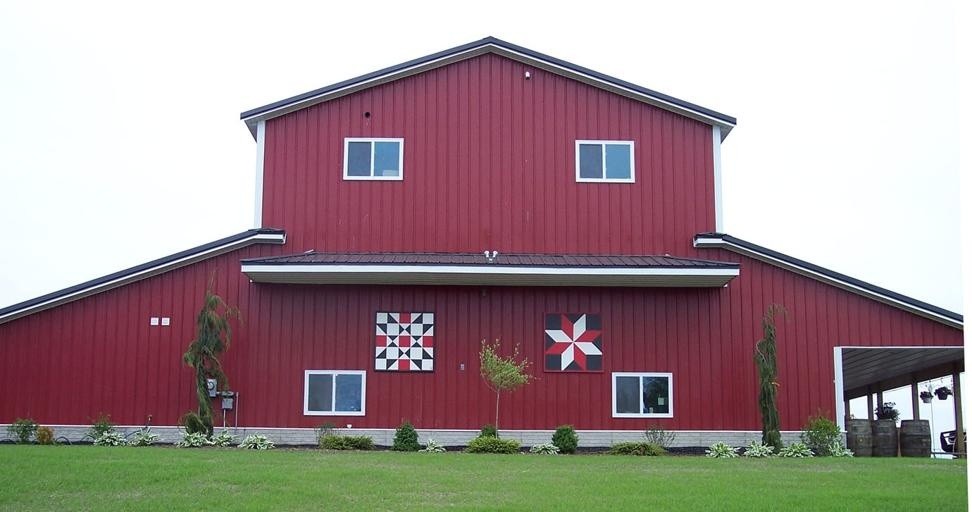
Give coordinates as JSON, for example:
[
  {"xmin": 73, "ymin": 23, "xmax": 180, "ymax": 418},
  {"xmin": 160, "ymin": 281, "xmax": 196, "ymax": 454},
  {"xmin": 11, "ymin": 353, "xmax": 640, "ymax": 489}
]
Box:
[
  {"xmin": 845, "ymin": 419, "xmax": 871, "ymax": 458},
  {"xmin": 900, "ymin": 420, "xmax": 931, "ymax": 458},
  {"xmin": 872, "ymin": 418, "xmax": 898, "ymax": 458}
]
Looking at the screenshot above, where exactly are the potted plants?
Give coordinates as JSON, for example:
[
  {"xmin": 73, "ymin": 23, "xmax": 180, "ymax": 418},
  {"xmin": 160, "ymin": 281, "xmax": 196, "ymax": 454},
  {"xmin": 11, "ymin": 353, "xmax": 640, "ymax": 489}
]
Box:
[
  {"xmin": 934, "ymin": 386, "xmax": 953, "ymax": 400},
  {"xmin": 920, "ymin": 391, "xmax": 933, "ymax": 403}
]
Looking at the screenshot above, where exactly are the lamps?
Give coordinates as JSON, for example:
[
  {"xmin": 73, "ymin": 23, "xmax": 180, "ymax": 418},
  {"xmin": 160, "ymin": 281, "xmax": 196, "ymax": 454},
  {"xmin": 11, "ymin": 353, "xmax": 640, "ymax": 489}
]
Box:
[{"xmin": 484, "ymin": 250, "xmax": 498, "ymax": 264}]
